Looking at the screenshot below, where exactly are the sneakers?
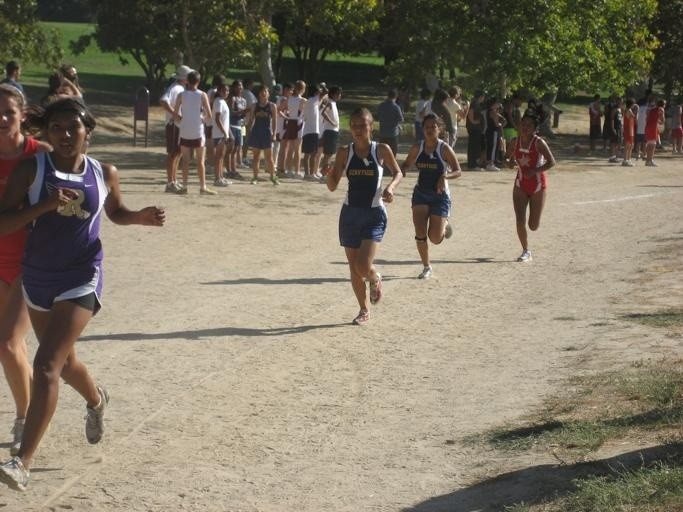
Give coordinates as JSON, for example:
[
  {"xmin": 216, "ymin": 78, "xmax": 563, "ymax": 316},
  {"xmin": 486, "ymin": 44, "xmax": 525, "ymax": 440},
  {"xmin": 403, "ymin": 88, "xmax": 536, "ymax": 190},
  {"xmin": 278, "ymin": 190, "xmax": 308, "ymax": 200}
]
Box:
[
  {"xmin": 164, "ymin": 180, "xmax": 188, "ymax": 195},
  {"xmin": 250, "ymin": 176, "xmax": 260, "ymax": 184},
  {"xmin": 199, "ymin": 187, "xmax": 219, "ymax": 196},
  {"xmin": 516, "ymin": 251, "xmax": 533, "ymax": 263},
  {"xmin": 470, "ymin": 160, "xmax": 502, "ymax": 173},
  {"xmin": 271, "ymin": 169, "xmax": 324, "ymax": 188},
  {"xmin": 214, "ymin": 170, "xmax": 244, "ymax": 187},
  {"xmin": 609, "ymin": 154, "xmax": 658, "ymax": 168},
  {"xmin": 369, "ymin": 275, "xmax": 382, "ymax": 305},
  {"xmin": 444, "ymin": 219, "xmax": 453, "ymax": 239},
  {"xmin": 0, "ymin": 458, "xmax": 30, "ymax": 493},
  {"xmin": 85, "ymin": 385, "xmax": 110, "ymax": 445},
  {"xmin": 418, "ymin": 266, "xmax": 434, "ymax": 280},
  {"xmin": 10, "ymin": 417, "xmax": 25, "ymax": 455},
  {"xmin": 352, "ymin": 309, "xmax": 370, "ymax": 325}
]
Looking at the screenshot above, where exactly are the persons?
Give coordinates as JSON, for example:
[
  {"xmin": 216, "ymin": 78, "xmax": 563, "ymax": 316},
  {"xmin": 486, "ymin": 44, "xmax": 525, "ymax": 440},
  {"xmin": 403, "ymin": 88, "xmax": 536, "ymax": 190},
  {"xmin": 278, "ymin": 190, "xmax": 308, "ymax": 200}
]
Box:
[
  {"xmin": 415, "ymin": 86, "xmax": 562, "ymax": 170},
  {"xmin": 158, "ymin": 66, "xmax": 342, "ymax": 194},
  {"xmin": 374, "ymin": 90, "xmax": 403, "ymax": 176},
  {"xmin": 1, "ymin": 60, "xmax": 91, "ymax": 197},
  {"xmin": 399, "ymin": 111, "xmax": 463, "ymax": 280},
  {"xmin": 589, "ymin": 86, "xmax": 683, "ymax": 166},
  {"xmin": 498, "ymin": 112, "xmax": 556, "ymax": 263},
  {"xmin": 0, "ymin": 96, "xmax": 166, "ymax": 492},
  {"xmin": 0, "ymin": 83, "xmax": 53, "ymax": 458},
  {"xmin": 325, "ymin": 105, "xmax": 404, "ymax": 325}
]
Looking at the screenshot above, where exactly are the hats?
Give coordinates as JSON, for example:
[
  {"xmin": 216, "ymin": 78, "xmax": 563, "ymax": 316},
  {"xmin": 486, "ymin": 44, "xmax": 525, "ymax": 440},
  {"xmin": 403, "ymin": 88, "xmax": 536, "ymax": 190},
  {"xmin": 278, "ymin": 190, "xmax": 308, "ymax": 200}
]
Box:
[{"xmin": 170, "ymin": 64, "xmax": 196, "ymax": 81}]
